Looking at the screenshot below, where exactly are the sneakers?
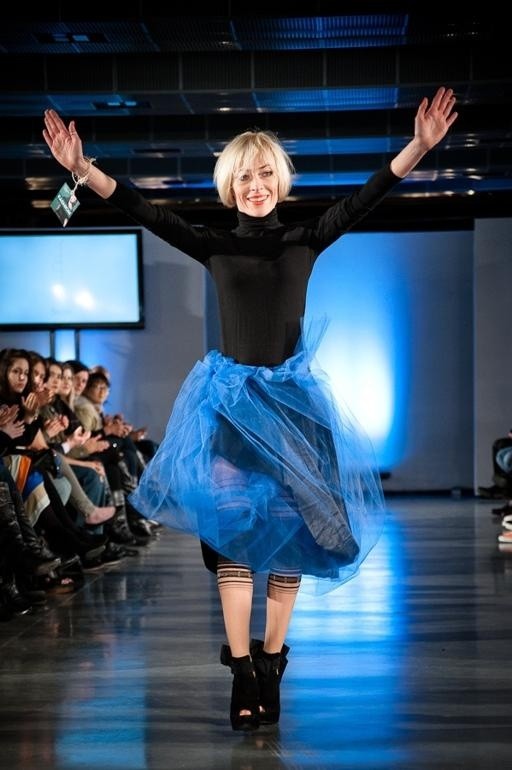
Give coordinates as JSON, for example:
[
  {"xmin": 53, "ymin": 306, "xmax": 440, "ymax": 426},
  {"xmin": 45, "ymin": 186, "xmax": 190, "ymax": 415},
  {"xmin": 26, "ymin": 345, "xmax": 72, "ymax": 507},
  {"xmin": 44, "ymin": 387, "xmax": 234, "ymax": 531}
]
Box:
[
  {"xmin": 492, "ymin": 503, "xmax": 511, "ymax": 544},
  {"xmin": 84, "ymin": 505, "xmax": 121, "ymax": 526}
]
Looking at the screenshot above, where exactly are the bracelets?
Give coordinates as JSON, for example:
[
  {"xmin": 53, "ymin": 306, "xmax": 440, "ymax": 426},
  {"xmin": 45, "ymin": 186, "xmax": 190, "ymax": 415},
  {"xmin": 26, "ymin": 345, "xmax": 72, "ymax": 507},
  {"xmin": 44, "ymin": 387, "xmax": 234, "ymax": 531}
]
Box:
[{"xmin": 72, "ymin": 153, "xmax": 96, "ymax": 185}]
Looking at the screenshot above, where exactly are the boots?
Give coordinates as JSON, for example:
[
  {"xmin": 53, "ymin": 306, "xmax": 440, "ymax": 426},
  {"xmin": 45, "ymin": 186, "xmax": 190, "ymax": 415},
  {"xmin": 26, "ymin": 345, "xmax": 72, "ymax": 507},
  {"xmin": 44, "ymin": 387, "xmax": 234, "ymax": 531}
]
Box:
[
  {"xmin": 110, "ymin": 488, "xmax": 149, "ymax": 546},
  {"xmin": 0, "ymin": 480, "xmax": 79, "ymax": 617}
]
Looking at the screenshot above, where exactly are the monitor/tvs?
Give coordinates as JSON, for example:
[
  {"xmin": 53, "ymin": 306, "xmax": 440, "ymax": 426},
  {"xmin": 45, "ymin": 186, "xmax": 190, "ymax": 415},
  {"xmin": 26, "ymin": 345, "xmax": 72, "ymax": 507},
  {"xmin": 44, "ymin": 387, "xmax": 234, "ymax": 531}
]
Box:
[{"xmin": 0, "ymin": 229, "xmax": 145, "ymax": 332}]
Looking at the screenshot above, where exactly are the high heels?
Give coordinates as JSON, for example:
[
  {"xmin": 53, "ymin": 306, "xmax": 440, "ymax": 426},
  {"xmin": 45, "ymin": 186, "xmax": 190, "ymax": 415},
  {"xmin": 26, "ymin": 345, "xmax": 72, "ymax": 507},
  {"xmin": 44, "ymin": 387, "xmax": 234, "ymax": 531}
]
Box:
[
  {"xmin": 252, "ymin": 638, "xmax": 289, "ymax": 725},
  {"xmin": 220, "ymin": 644, "xmax": 260, "ymax": 732}
]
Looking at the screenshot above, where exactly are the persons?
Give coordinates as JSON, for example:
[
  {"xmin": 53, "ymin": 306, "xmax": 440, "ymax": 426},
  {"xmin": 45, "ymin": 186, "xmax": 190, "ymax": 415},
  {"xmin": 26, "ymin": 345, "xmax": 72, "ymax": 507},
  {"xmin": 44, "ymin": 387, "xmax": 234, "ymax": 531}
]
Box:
[
  {"xmin": 490, "ymin": 427, "xmax": 512, "ymax": 545},
  {"xmin": 39, "ymin": 86, "xmax": 459, "ymax": 731},
  {"xmin": 1, "ymin": 349, "xmax": 157, "ymax": 624}
]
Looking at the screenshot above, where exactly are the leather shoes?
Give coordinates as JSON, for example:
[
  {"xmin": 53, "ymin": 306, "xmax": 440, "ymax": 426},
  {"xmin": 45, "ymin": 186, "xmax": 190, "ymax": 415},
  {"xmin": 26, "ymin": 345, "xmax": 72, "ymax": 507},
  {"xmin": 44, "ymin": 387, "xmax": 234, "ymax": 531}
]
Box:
[
  {"xmin": 476, "ymin": 483, "xmax": 512, "ymax": 500},
  {"xmin": 81, "ymin": 534, "xmax": 139, "ymax": 574}
]
